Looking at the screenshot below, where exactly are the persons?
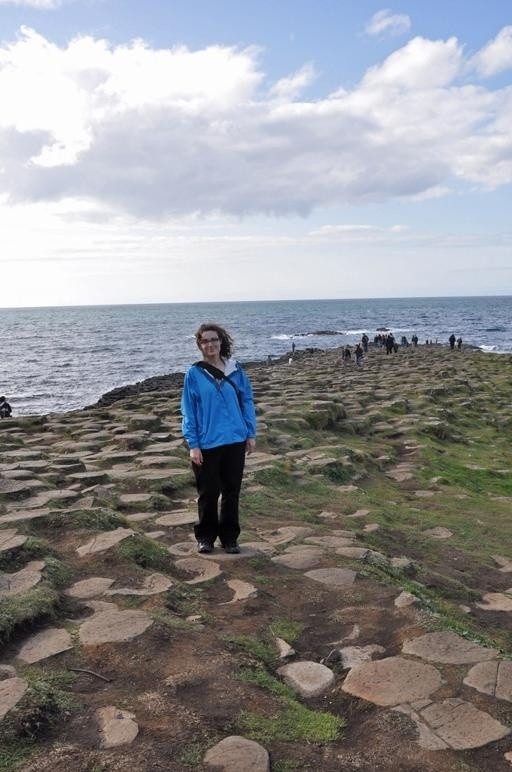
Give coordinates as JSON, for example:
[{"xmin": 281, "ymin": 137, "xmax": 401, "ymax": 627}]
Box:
[
  {"xmin": 288, "ymin": 355, "xmax": 293, "ymax": 365},
  {"xmin": 265, "ymin": 354, "xmax": 273, "ymax": 366},
  {"xmin": 340, "ymin": 329, "xmax": 434, "ymax": 370},
  {"xmin": 448, "ymin": 333, "xmax": 456, "ymax": 349},
  {"xmin": 179, "ymin": 321, "xmax": 257, "ymax": 554},
  {"xmin": 0, "ymin": 395, "xmax": 13, "ymax": 420},
  {"xmin": 456, "ymin": 336, "xmax": 463, "ymax": 349},
  {"xmin": 290, "ymin": 341, "xmax": 296, "ymax": 357}
]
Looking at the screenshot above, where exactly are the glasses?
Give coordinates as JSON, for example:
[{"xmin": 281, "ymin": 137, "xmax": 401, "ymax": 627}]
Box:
[{"xmin": 200, "ymin": 337, "xmax": 219, "ymax": 344}]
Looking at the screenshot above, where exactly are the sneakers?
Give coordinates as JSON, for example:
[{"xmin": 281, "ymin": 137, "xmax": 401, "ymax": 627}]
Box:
[
  {"xmin": 220, "ymin": 541, "xmax": 241, "ymax": 554},
  {"xmin": 198, "ymin": 539, "xmax": 214, "ymax": 553}
]
